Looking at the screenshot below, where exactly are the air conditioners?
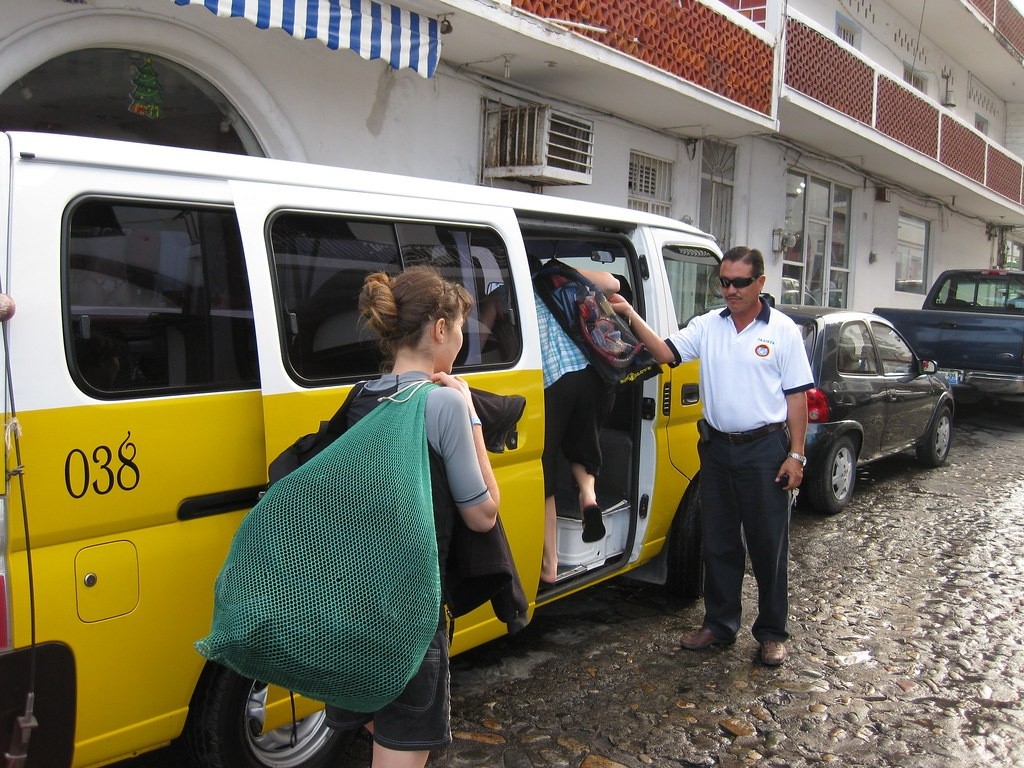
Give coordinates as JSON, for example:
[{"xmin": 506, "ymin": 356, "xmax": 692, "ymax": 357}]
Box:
[{"xmin": 498, "ymin": 107, "xmax": 594, "ymax": 186}]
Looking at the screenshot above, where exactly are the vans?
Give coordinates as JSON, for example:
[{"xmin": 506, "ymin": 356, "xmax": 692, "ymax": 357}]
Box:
[{"xmin": 0, "ymin": 131, "xmax": 774, "ymax": 768}]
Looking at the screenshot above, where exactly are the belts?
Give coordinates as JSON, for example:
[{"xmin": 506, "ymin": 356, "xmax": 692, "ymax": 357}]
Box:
[{"xmin": 708, "ymin": 422, "xmax": 785, "ymax": 445}]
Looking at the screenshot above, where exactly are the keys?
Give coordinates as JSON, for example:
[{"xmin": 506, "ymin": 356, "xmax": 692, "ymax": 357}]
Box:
[{"xmin": 791, "ymin": 487, "xmax": 800, "ymax": 506}]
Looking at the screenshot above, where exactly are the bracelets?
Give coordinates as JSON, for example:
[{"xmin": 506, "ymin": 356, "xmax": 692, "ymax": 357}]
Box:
[{"xmin": 627, "ymin": 304, "xmax": 632, "ymax": 327}]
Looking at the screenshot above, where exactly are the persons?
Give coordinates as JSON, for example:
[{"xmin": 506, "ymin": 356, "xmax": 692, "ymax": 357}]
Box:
[
  {"xmin": 608, "ymin": 247, "xmax": 815, "ymax": 667},
  {"xmin": 474, "ymin": 253, "xmax": 620, "ymax": 590},
  {"xmin": 324, "ymin": 264, "xmax": 499, "ymax": 768}
]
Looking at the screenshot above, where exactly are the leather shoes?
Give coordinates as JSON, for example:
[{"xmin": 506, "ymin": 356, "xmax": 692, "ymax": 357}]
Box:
[
  {"xmin": 760, "ymin": 639, "xmax": 786, "ymax": 665},
  {"xmin": 679, "ymin": 624, "xmax": 736, "ymax": 649}
]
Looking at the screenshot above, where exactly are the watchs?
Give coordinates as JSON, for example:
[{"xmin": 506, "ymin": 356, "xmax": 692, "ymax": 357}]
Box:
[{"xmin": 788, "ymin": 452, "xmax": 807, "ymax": 466}]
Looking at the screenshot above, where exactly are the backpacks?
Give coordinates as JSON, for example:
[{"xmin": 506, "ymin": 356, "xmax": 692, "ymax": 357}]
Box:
[{"xmin": 269, "ymin": 380, "xmax": 365, "ymax": 486}]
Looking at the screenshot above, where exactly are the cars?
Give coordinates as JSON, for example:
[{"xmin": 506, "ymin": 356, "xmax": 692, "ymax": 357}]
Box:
[{"xmin": 681, "ymin": 304, "xmax": 954, "ymax": 513}]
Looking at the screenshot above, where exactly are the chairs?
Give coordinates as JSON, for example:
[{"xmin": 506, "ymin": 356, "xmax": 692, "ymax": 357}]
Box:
[
  {"xmin": 433, "ymin": 255, "xmax": 485, "ymax": 300},
  {"xmin": 607, "ymin": 275, "xmax": 633, "ymax": 431},
  {"xmin": 839, "ymin": 337, "xmax": 855, "ymax": 369}
]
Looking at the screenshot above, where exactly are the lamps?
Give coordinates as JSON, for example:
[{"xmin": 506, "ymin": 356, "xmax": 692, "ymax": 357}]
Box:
[{"xmin": 942, "ymin": 90, "xmax": 956, "ymax": 111}]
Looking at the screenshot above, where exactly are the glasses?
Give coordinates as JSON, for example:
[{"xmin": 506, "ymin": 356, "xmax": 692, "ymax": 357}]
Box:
[{"xmin": 718, "ymin": 274, "xmax": 761, "ymax": 289}]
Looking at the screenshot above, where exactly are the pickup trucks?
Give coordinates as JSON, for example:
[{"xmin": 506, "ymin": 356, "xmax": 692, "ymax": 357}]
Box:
[{"xmin": 872, "ymin": 269, "xmax": 1024, "ymax": 426}]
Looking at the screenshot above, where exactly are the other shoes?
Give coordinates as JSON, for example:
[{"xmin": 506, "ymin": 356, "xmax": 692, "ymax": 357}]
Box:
[
  {"xmin": 582, "ymin": 504, "xmax": 606, "ymax": 543},
  {"xmin": 539, "ymin": 577, "xmax": 556, "ymax": 589}
]
